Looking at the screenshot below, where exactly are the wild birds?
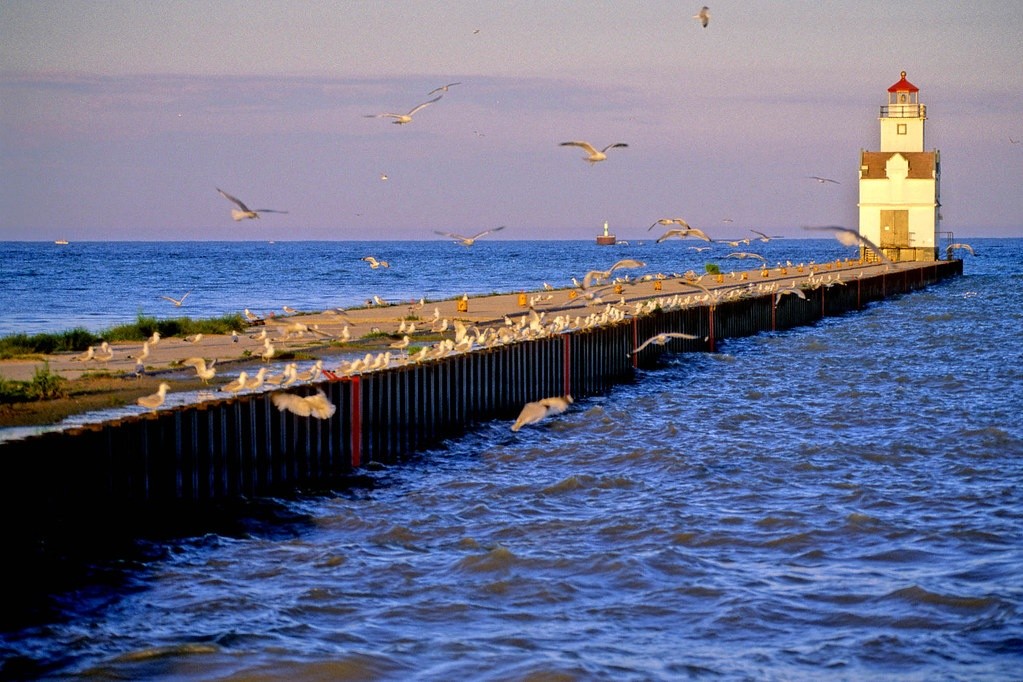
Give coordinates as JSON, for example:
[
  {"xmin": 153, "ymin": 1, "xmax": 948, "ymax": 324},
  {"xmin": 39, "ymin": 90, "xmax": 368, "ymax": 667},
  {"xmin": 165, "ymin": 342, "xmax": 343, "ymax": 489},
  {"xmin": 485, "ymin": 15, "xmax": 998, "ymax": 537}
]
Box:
[
  {"xmin": 947, "ymin": 243, "xmax": 974, "ymax": 256},
  {"xmin": 726, "ymin": 251, "xmax": 765, "ymax": 261},
  {"xmin": 511, "ymin": 395, "xmax": 573, "ymax": 431},
  {"xmin": 714, "ymin": 230, "xmax": 786, "ymax": 248},
  {"xmin": 692, "ymin": 6, "xmax": 711, "ymax": 28},
  {"xmin": 365, "ymin": 94, "xmax": 442, "ymax": 125},
  {"xmin": 648, "ymin": 218, "xmax": 691, "ymax": 233},
  {"xmin": 71, "ymin": 258, "xmax": 864, "ymax": 397},
  {"xmin": 215, "ymin": 188, "xmax": 286, "ymax": 222},
  {"xmin": 616, "ymin": 241, "xmax": 629, "ymax": 246},
  {"xmin": 430, "ymin": 82, "xmax": 461, "ymax": 95},
  {"xmin": 657, "ymin": 229, "xmax": 712, "ymax": 244},
  {"xmin": 434, "ymin": 228, "xmax": 503, "ymax": 247},
  {"xmin": 806, "ymin": 176, "xmax": 842, "ymax": 184},
  {"xmin": 561, "ymin": 141, "xmax": 629, "ymax": 166},
  {"xmin": 361, "ymin": 256, "xmax": 388, "ymax": 270},
  {"xmin": 271, "ymin": 386, "xmax": 337, "ymax": 420},
  {"xmin": 688, "ymin": 247, "xmax": 712, "ymax": 253},
  {"xmin": 137, "ymin": 382, "xmax": 172, "ymax": 416},
  {"xmin": 382, "ymin": 174, "xmax": 387, "ymax": 180}
]
